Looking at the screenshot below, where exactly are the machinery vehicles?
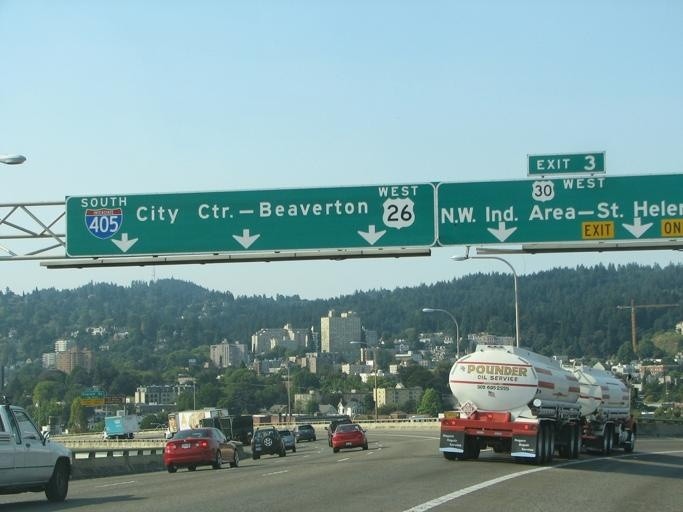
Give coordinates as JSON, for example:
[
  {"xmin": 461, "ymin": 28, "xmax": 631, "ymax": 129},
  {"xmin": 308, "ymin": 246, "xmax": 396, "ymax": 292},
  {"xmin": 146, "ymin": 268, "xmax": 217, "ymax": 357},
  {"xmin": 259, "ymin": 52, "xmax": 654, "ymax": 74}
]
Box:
[{"xmin": 436, "ymin": 344, "xmax": 637, "ymax": 465}]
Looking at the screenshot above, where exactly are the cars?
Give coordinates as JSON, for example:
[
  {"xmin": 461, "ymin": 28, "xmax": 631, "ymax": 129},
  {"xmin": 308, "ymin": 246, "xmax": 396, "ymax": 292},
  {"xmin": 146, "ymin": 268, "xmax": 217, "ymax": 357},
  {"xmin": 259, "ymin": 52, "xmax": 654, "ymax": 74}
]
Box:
[
  {"xmin": 164, "ymin": 428, "xmax": 240, "ymax": 473},
  {"xmin": 251, "ymin": 414, "xmax": 369, "ymax": 463}
]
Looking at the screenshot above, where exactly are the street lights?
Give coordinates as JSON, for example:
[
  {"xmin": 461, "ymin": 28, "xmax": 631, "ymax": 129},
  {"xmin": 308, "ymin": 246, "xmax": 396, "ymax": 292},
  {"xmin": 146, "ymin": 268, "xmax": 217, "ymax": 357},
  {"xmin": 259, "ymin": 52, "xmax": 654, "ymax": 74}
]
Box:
[
  {"xmin": 0, "ymin": 154, "xmax": 28, "ymax": 165},
  {"xmin": 449, "ymin": 255, "xmax": 519, "ymax": 349},
  {"xmin": 92, "ymin": 385, "xmax": 107, "ymax": 417},
  {"xmin": 422, "ymin": 308, "xmax": 460, "ymax": 359},
  {"xmin": 350, "ymin": 340, "xmax": 378, "ymax": 422},
  {"xmin": 280, "ymin": 363, "xmax": 291, "ymax": 415},
  {"xmin": 178, "ymin": 373, "xmax": 196, "ymax": 410}
]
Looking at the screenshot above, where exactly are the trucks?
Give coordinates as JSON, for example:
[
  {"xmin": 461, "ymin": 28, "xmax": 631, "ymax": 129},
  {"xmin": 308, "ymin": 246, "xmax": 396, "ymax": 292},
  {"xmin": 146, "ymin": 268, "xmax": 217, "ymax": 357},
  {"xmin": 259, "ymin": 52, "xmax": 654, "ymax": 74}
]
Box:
[
  {"xmin": 101, "ymin": 413, "xmax": 139, "ymax": 439},
  {"xmin": 162, "ymin": 407, "xmax": 254, "ymax": 446}
]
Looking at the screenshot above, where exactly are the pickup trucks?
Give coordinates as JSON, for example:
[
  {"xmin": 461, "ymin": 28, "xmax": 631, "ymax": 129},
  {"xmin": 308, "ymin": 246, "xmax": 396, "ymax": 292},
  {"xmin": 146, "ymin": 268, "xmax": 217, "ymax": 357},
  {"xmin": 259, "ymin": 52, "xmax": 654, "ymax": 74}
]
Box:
[{"xmin": 0, "ymin": 396, "xmax": 74, "ymax": 504}]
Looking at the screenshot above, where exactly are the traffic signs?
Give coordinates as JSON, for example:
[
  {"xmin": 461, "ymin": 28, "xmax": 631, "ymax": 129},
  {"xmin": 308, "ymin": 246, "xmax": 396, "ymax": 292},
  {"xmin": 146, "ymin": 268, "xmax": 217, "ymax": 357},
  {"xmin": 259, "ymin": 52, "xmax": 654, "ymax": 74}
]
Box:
[
  {"xmin": 64, "ymin": 184, "xmax": 435, "ymax": 255},
  {"xmin": 435, "ymin": 174, "xmax": 682, "ymax": 245}
]
